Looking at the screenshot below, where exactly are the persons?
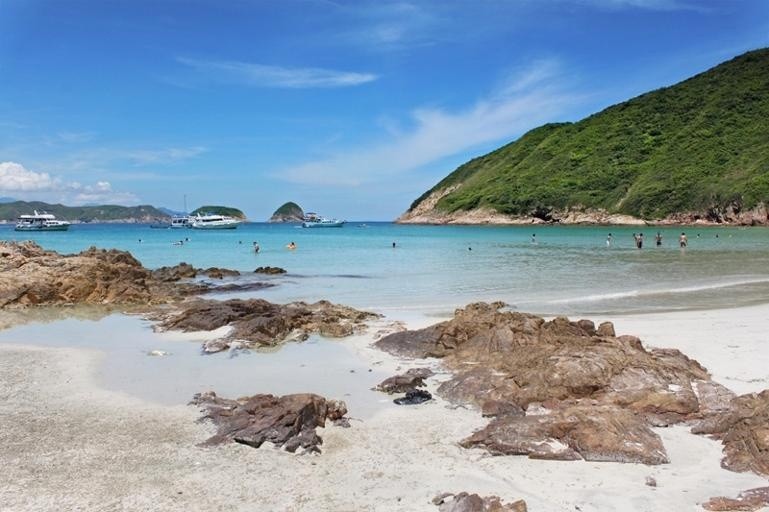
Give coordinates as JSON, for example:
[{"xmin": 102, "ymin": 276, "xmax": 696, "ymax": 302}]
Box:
[
  {"xmin": 604, "ymin": 229, "xmax": 721, "ymax": 248},
  {"xmin": 284, "ymin": 241, "xmax": 295, "ymax": 248},
  {"xmin": 252, "ymin": 241, "xmax": 260, "ymax": 253},
  {"xmin": 529, "ymin": 233, "xmax": 536, "ymax": 244}
]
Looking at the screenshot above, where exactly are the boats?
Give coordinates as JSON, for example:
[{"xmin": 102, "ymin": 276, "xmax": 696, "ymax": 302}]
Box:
[
  {"xmin": 14, "ymin": 208, "xmax": 72, "ymax": 232},
  {"xmin": 149, "ymin": 213, "xmax": 242, "ymax": 229},
  {"xmin": 357, "ymin": 223, "xmax": 367, "ymax": 227},
  {"xmin": 301, "ymin": 217, "xmax": 348, "ymax": 228}
]
